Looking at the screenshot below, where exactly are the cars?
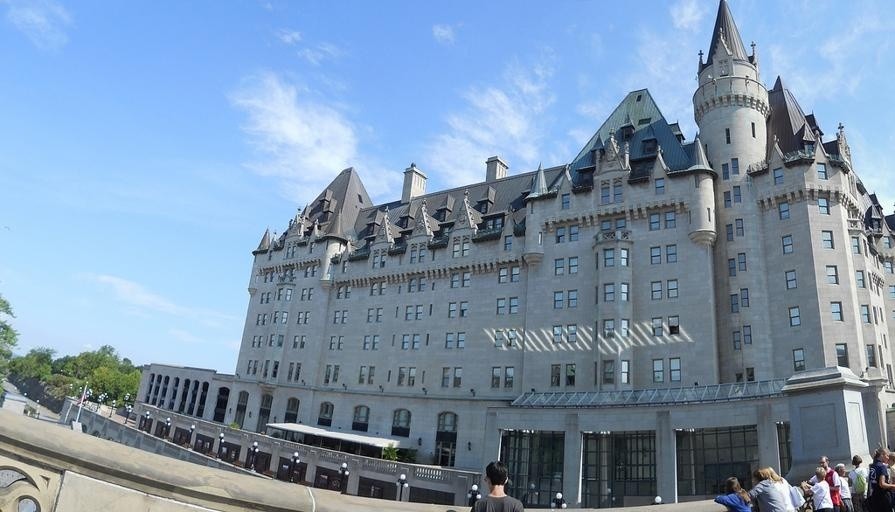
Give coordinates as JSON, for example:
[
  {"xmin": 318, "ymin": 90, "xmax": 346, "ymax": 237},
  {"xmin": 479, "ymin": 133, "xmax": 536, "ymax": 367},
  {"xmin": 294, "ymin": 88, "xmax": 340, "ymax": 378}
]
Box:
[
  {"xmin": 281, "ymin": 464, "xmax": 342, "ymax": 491},
  {"xmin": 197, "ymin": 440, "xmax": 256, "ymax": 467}
]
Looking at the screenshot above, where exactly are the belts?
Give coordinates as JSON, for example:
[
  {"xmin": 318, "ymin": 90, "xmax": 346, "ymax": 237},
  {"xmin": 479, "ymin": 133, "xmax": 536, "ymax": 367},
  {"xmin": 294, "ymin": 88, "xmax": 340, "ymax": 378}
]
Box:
[{"xmin": 841, "ymin": 499, "xmax": 851, "ymax": 501}]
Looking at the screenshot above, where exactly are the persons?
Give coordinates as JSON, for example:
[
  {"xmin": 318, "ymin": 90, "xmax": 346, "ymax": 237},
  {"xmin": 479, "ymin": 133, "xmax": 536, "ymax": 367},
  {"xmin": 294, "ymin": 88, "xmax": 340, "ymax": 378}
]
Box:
[
  {"xmin": 470, "ymin": 461, "xmax": 524, "ymax": 512},
  {"xmin": 714, "ymin": 447, "xmax": 895, "ymax": 512}
]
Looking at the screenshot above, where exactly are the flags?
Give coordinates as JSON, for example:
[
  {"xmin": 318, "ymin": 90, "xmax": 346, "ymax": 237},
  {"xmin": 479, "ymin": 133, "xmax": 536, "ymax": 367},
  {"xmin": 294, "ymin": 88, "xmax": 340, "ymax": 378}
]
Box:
[{"xmin": 76, "ymin": 386, "xmax": 90, "ymax": 406}]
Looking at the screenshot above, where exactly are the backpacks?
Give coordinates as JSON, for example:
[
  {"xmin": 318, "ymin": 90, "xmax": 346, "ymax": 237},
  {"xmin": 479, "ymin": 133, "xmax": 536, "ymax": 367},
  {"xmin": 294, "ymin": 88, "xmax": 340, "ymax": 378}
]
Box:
[{"xmin": 852, "ymin": 469, "xmax": 865, "ymax": 494}]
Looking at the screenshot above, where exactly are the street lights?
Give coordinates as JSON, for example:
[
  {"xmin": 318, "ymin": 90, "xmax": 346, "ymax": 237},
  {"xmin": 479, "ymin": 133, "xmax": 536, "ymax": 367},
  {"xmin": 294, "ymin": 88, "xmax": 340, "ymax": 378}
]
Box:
[
  {"xmin": 467, "ymin": 484, "xmax": 482, "ymax": 506},
  {"xmin": 215, "ymin": 432, "xmax": 225, "ymax": 458},
  {"xmin": 337, "ymin": 462, "xmax": 350, "ymax": 492},
  {"xmin": 396, "ymin": 473, "xmax": 408, "ymax": 500},
  {"xmin": 249, "ymin": 442, "xmax": 260, "ymax": 471},
  {"xmin": 526, "ymin": 484, "xmax": 539, "ymax": 504},
  {"xmin": 551, "ymin": 492, "xmax": 567, "ymax": 509},
  {"xmin": 603, "ymin": 488, "xmax": 616, "ymax": 507},
  {"xmin": 651, "ymin": 495, "xmax": 666, "ymax": 505},
  {"xmin": 2, "ymin": 369, "xmax": 195, "ymax": 449},
  {"xmin": 288, "ymin": 451, "xmax": 300, "ymax": 482}
]
